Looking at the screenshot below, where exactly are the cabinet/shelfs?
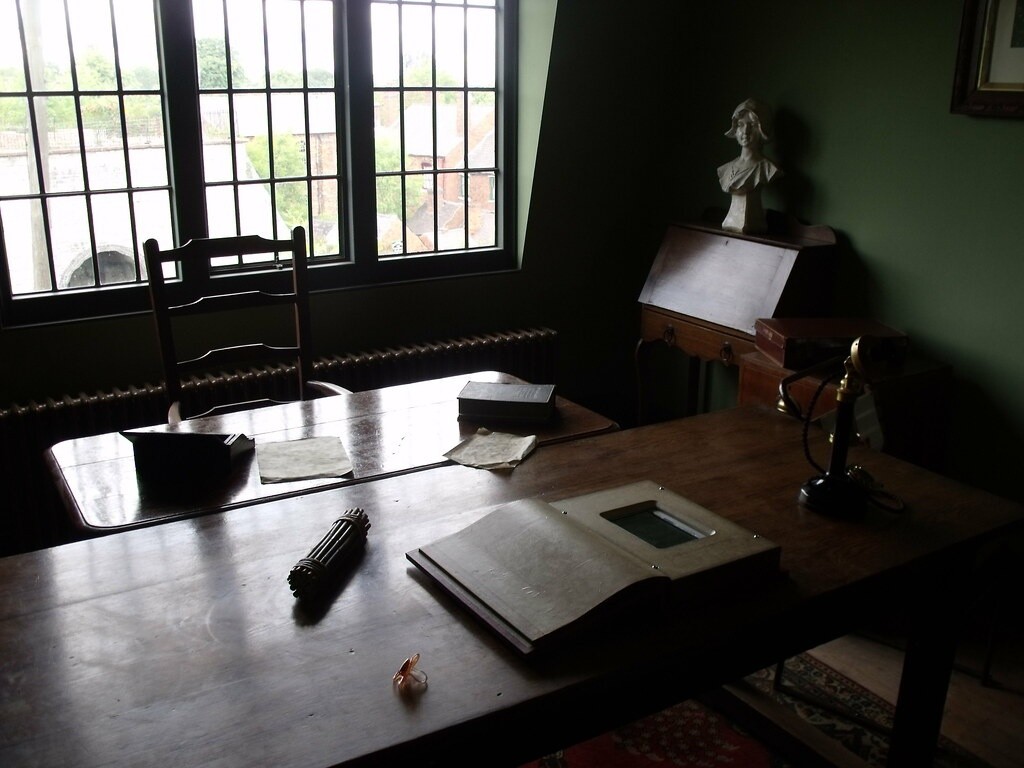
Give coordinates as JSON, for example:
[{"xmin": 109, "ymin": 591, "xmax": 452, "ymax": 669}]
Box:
[
  {"xmin": 634, "ymin": 208, "xmax": 842, "ymax": 426},
  {"xmin": 737, "ymin": 352, "xmax": 954, "ymax": 474}
]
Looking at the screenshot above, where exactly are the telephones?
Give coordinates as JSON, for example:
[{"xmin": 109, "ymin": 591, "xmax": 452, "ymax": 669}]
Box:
[{"xmin": 777, "ymin": 335, "xmax": 912, "ymax": 528}]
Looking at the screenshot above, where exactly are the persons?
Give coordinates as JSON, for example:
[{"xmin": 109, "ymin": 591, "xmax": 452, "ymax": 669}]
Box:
[{"xmin": 717, "ymin": 99, "xmax": 783, "ymax": 193}]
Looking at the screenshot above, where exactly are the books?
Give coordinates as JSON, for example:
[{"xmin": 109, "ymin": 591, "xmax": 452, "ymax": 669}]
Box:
[
  {"xmin": 405, "ymin": 479, "xmax": 782, "ymax": 658},
  {"xmin": 457, "ymin": 381, "xmax": 556, "ymax": 427}
]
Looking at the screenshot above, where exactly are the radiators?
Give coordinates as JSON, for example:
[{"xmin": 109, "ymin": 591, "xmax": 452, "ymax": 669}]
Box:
[{"xmin": 0, "ymin": 326, "xmax": 560, "ymax": 555}]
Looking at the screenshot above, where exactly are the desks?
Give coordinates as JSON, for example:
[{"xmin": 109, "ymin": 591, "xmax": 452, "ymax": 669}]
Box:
[
  {"xmin": 0, "ymin": 400, "xmax": 1024, "ymax": 768},
  {"xmin": 41, "ymin": 366, "xmax": 620, "ymax": 536}
]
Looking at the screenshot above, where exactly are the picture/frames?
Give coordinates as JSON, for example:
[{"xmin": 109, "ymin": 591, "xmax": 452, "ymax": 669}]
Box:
[{"xmin": 951, "ymin": 0, "xmax": 1024, "ymax": 119}]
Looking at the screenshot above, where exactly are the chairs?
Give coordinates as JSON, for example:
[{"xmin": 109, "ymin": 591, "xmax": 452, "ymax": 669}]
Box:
[{"xmin": 143, "ymin": 225, "xmax": 355, "ymax": 426}]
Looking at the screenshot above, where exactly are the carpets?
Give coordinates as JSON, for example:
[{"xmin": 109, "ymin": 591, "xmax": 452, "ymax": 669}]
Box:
[{"xmin": 516, "ymin": 652, "xmax": 996, "ymax": 768}]
